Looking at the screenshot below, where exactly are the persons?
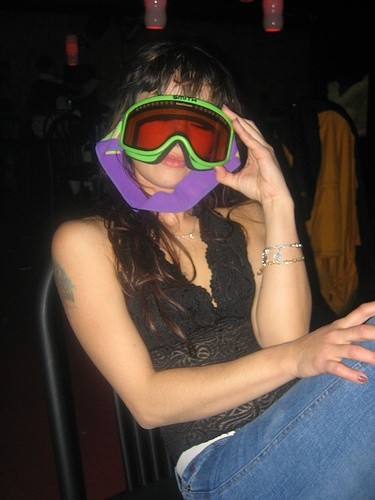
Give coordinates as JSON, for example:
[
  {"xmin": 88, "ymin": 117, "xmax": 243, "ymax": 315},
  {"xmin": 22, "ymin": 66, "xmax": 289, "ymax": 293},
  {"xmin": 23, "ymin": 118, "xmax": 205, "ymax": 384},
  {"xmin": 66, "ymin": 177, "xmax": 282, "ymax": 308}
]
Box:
[
  {"xmin": 21, "ymin": 20, "xmax": 274, "ymax": 211},
  {"xmin": 51, "ymin": 34, "xmax": 375, "ymax": 500}
]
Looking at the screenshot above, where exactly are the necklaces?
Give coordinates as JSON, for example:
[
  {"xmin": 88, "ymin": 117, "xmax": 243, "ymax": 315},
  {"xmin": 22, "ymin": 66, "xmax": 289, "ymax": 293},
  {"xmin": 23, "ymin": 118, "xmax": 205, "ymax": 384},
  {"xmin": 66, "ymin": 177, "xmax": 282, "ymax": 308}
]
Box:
[{"xmin": 170, "ymin": 216, "xmax": 201, "ymax": 241}]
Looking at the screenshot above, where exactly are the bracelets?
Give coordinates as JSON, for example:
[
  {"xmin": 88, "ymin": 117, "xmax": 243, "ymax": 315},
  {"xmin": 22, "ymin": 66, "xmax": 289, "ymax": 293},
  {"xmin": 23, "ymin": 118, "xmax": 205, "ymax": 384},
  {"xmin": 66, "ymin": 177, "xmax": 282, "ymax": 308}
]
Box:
[{"xmin": 260, "ymin": 240, "xmax": 306, "ymax": 268}]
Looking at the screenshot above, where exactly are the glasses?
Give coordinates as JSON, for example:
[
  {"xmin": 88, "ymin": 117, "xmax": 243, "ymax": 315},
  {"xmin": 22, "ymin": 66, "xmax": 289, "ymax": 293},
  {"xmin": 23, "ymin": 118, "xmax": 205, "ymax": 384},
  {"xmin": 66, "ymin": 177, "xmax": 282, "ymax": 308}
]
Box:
[{"xmin": 96, "ymin": 95, "xmax": 241, "ymax": 212}]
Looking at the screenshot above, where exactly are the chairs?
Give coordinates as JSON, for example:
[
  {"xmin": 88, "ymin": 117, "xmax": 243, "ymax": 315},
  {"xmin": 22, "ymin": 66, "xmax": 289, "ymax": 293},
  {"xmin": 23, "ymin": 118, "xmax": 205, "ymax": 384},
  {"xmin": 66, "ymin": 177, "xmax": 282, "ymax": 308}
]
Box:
[{"xmin": 37, "ymin": 258, "xmax": 183, "ymax": 500}]
